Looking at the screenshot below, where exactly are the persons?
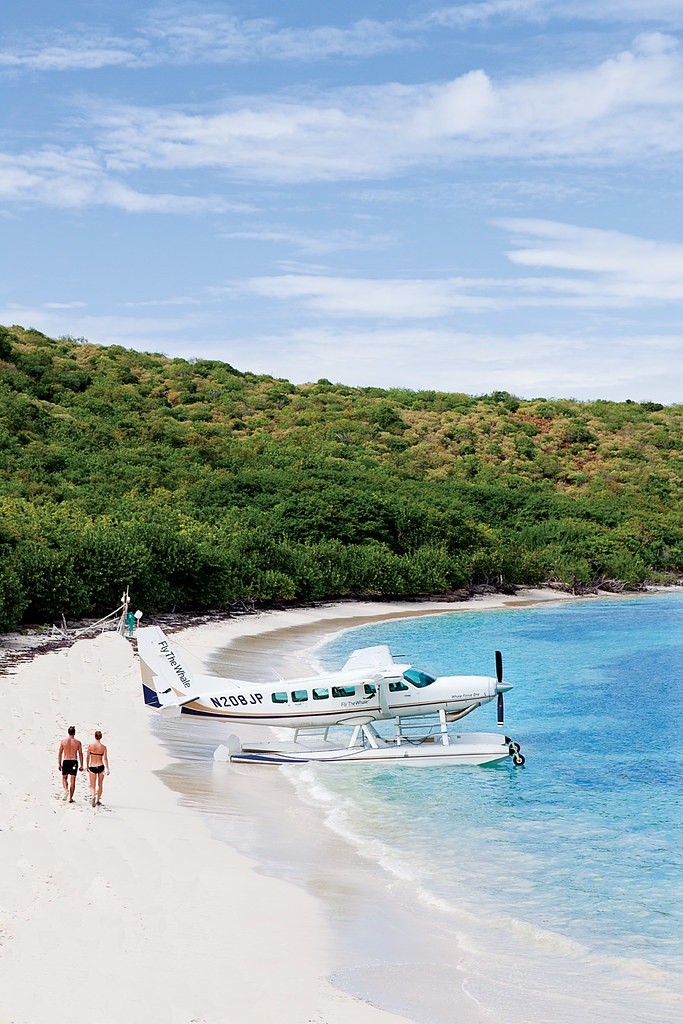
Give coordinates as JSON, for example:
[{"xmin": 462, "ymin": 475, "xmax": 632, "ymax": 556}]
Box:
[
  {"xmin": 86, "ymin": 731, "xmax": 110, "ymax": 807},
  {"xmin": 57, "ymin": 726, "xmax": 83, "ymax": 804}
]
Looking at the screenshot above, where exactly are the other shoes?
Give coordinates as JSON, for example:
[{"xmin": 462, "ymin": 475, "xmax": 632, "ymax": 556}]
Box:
[
  {"xmin": 92, "ymin": 798, "xmax": 96, "ymax": 807},
  {"xmin": 62, "ymin": 792, "xmax": 69, "ymax": 800},
  {"xmin": 97, "ymin": 801, "xmax": 101, "ymax": 805},
  {"xmin": 69, "ymin": 800, "xmax": 75, "ymax": 803}
]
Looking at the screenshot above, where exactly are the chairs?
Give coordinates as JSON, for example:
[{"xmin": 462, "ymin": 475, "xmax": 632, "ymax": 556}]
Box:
[
  {"xmin": 364, "ymin": 684, "xmax": 373, "ymax": 694},
  {"xmin": 389, "ymin": 683, "xmax": 395, "ymax": 692}
]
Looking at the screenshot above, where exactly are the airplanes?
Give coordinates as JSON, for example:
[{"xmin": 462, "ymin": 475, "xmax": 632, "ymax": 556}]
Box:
[{"xmin": 134, "ymin": 626, "xmax": 527, "ymax": 767}]
